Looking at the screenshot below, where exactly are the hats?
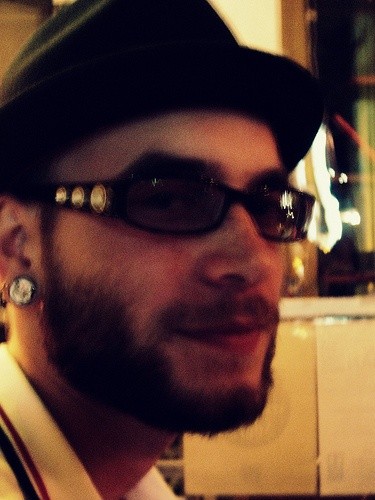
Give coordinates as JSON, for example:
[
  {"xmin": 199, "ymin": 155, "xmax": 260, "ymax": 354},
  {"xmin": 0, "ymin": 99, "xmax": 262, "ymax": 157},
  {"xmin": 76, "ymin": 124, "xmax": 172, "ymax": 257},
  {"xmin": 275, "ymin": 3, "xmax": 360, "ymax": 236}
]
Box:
[{"xmin": 0, "ymin": 0, "xmax": 326, "ymax": 192}]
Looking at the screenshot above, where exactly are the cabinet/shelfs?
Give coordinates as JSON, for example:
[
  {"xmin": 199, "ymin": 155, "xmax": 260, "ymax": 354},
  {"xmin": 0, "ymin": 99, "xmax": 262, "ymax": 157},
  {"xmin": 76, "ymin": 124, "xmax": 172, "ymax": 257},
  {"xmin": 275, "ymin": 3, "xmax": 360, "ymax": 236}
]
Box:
[{"xmin": 183, "ymin": 317, "xmax": 375, "ymax": 500}]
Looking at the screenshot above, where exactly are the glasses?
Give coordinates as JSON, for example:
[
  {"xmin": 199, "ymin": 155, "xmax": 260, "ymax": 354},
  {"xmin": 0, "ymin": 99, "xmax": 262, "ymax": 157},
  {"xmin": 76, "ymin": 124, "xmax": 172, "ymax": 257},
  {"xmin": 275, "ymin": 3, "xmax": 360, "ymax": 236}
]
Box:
[{"xmin": 46, "ymin": 156, "xmax": 317, "ymax": 243}]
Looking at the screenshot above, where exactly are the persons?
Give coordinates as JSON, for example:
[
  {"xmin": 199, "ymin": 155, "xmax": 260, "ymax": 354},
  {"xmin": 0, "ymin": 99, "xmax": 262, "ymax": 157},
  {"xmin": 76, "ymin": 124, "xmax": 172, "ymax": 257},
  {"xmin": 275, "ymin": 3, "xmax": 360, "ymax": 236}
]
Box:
[{"xmin": 0, "ymin": 0, "xmax": 328, "ymax": 500}]
